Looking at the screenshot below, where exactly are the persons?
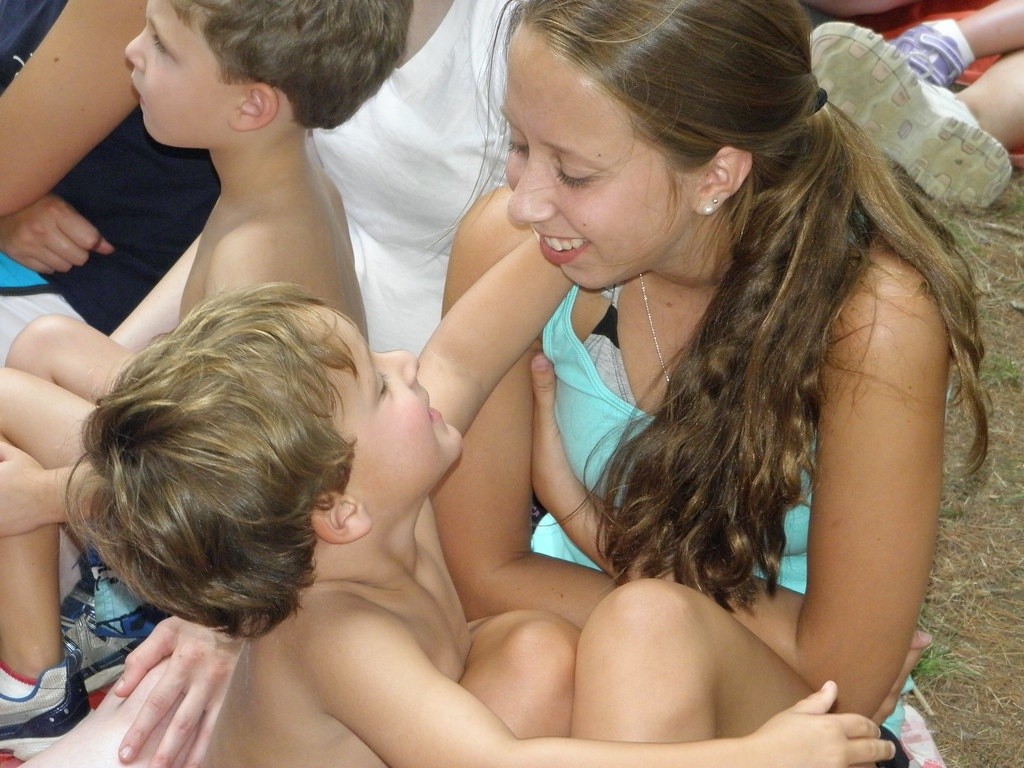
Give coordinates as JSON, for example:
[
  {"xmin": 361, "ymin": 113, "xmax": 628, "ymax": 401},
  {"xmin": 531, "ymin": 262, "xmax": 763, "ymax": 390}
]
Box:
[
  {"xmin": 0, "ymin": 0, "xmax": 222, "ymax": 334},
  {"xmin": 804, "ymin": 0, "xmax": 1024, "ymax": 208},
  {"xmin": 0, "ymin": 0, "xmax": 414, "ymax": 753},
  {"xmin": 414, "ymin": 1, "xmax": 988, "ymax": 744},
  {"xmin": 25, "ymin": 0, "xmax": 510, "ymax": 768},
  {"xmin": 81, "ymin": 280, "xmax": 896, "ymax": 768}
]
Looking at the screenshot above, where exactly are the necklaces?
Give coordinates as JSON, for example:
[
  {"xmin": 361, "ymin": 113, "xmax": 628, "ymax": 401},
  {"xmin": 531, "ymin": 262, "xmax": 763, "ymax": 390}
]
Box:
[{"xmin": 640, "ymin": 273, "xmax": 669, "ymax": 381}]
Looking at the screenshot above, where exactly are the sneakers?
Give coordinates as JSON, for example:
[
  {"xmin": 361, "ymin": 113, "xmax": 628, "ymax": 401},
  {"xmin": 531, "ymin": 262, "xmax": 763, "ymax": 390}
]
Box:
[
  {"xmin": 885, "ymin": 22, "xmax": 966, "ymax": 92},
  {"xmin": 808, "ymin": 22, "xmax": 1012, "ymax": 214},
  {"xmin": 62, "ymin": 547, "xmax": 171, "ymax": 692},
  {"xmin": 0, "ymin": 650, "xmax": 96, "ymax": 762}
]
